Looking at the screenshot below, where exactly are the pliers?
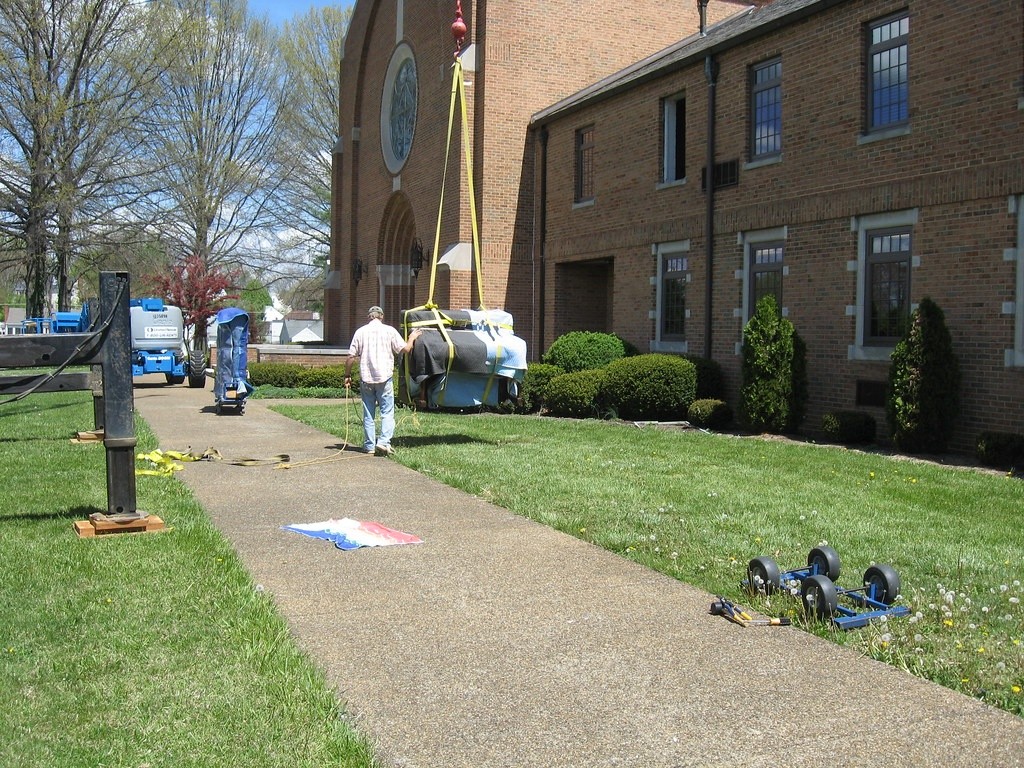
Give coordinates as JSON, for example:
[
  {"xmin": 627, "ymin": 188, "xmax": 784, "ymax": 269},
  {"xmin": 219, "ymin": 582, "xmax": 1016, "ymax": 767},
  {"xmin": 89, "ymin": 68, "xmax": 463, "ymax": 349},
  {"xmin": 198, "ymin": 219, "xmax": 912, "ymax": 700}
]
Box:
[{"xmin": 716, "ymin": 595, "xmax": 752, "ymax": 626}]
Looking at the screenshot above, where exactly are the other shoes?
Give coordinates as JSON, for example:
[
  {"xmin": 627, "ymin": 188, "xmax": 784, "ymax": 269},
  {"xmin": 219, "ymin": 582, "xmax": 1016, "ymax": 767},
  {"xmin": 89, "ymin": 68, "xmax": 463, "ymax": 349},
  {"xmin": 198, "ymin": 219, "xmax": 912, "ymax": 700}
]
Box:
[
  {"xmin": 375, "ymin": 444, "xmax": 395, "ymax": 455},
  {"xmin": 363, "ymin": 450, "xmax": 376, "ymax": 453}
]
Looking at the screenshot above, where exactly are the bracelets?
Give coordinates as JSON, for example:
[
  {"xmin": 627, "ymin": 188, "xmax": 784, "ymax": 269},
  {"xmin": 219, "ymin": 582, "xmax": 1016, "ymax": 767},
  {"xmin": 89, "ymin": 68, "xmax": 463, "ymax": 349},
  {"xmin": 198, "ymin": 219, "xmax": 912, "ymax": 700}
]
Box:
[{"xmin": 345, "ymin": 373, "xmax": 351, "ymax": 378}]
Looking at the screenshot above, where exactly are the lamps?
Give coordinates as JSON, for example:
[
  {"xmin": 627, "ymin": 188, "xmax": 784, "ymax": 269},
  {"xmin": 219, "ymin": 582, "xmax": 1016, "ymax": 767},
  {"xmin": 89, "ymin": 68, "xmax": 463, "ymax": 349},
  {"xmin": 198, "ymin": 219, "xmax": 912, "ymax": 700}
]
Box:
[
  {"xmin": 409, "ymin": 237, "xmax": 430, "ymax": 279},
  {"xmin": 352, "ymin": 257, "xmax": 368, "ymax": 286}
]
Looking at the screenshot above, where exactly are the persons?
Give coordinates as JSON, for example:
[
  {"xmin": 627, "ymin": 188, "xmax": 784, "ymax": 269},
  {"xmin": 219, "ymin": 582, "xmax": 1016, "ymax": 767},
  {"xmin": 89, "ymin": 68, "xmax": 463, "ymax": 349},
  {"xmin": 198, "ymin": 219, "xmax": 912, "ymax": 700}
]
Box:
[{"xmin": 344, "ymin": 306, "xmax": 422, "ymax": 454}]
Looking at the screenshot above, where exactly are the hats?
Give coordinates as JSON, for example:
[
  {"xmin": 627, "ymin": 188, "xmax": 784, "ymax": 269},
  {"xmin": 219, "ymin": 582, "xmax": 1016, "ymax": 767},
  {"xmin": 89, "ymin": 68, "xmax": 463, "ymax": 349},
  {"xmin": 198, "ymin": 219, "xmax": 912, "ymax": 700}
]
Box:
[{"xmin": 368, "ymin": 306, "xmax": 384, "ymax": 315}]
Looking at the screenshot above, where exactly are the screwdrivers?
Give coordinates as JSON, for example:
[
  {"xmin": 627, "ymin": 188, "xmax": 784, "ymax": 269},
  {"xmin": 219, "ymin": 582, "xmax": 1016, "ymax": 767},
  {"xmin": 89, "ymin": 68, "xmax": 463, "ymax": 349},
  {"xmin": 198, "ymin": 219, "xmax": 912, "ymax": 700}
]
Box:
[{"xmin": 743, "ymin": 617, "xmax": 791, "ymax": 626}]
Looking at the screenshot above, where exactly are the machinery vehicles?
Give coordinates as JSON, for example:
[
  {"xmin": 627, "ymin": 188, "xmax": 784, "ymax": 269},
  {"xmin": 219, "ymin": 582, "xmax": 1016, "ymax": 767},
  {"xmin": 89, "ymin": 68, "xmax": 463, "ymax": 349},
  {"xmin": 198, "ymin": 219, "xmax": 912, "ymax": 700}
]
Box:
[
  {"xmin": 128, "ymin": 296, "xmax": 206, "ymax": 389},
  {"xmin": 0, "ymin": 301, "xmax": 91, "ymax": 334}
]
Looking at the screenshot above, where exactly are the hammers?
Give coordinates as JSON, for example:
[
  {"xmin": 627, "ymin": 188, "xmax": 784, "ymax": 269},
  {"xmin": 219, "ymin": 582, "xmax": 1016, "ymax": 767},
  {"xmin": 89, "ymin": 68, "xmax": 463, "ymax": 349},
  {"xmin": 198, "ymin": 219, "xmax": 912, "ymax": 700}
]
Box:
[{"xmin": 711, "ymin": 602, "xmax": 749, "ymax": 628}]
